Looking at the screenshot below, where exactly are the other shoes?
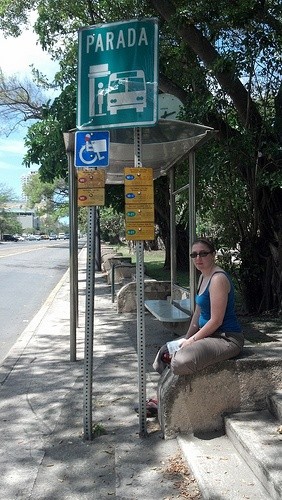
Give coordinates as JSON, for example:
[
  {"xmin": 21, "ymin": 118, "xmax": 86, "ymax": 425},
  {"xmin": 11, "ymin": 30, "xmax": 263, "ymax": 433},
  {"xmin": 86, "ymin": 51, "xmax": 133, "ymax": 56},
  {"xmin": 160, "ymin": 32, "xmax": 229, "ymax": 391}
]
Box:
[{"xmin": 135, "ymin": 399, "xmax": 157, "ymax": 417}]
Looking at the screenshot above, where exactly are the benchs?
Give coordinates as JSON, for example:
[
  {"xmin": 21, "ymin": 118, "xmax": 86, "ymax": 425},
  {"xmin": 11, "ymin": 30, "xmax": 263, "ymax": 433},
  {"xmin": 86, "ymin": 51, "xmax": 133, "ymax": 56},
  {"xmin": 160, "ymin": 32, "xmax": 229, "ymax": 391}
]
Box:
[{"xmin": 145, "ymin": 300, "xmax": 192, "ymax": 322}]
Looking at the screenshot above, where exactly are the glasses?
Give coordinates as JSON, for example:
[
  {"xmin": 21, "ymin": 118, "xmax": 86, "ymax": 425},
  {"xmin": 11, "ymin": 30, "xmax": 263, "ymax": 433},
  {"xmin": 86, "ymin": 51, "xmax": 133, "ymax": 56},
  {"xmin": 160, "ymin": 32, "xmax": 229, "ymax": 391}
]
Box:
[{"xmin": 189, "ymin": 251, "xmax": 211, "ymax": 258}]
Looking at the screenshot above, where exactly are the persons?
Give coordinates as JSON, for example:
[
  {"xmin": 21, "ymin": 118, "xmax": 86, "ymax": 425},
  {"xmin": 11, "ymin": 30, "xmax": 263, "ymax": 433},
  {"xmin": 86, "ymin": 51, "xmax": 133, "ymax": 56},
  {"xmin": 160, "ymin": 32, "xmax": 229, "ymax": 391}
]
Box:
[{"xmin": 133, "ymin": 236, "xmax": 245, "ymax": 420}]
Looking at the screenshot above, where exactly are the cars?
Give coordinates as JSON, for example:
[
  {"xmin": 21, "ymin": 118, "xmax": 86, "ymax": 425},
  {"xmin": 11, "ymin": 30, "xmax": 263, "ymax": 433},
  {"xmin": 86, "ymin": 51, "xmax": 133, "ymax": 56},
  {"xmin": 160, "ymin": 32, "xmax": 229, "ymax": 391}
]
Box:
[{"xmin": 3, "ymin": 230, "xmax": 82, "ymax": 242}]
